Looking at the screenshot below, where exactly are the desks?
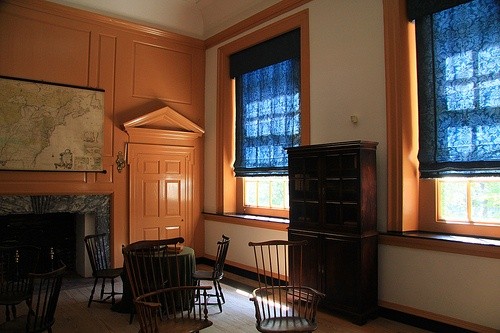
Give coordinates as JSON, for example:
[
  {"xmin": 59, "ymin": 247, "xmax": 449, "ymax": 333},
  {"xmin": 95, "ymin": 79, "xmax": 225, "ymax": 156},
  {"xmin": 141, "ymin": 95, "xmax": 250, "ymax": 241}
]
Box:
[{"xmin": 122, "ymin": 246, "xmax": 197, "ymax": 318}]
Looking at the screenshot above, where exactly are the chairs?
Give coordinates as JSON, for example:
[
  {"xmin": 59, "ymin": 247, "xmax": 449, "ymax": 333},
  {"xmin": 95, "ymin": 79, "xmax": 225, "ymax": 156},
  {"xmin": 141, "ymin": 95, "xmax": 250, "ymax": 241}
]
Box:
[
  {"xmin": 113, "ymin": 234, "xmax": 230, "ymax": 333},
  {"xmin": 249, "ymin": 239, "xmax": 325, "ymax": 333},
  {"xmin": 84, "ymin": 232, "xmax": 123, "ymax": 307},
  {"xmin": 0, "ymin": 240, "xmax": 66, "ymax": 333}
]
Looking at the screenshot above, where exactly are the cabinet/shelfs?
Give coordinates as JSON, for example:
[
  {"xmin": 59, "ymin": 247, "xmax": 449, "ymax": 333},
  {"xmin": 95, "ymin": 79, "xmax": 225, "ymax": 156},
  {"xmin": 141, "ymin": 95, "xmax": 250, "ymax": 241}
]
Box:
[{"xmin": 282, "ymin": 141, "xmax": 381, "ymax": 326}]
